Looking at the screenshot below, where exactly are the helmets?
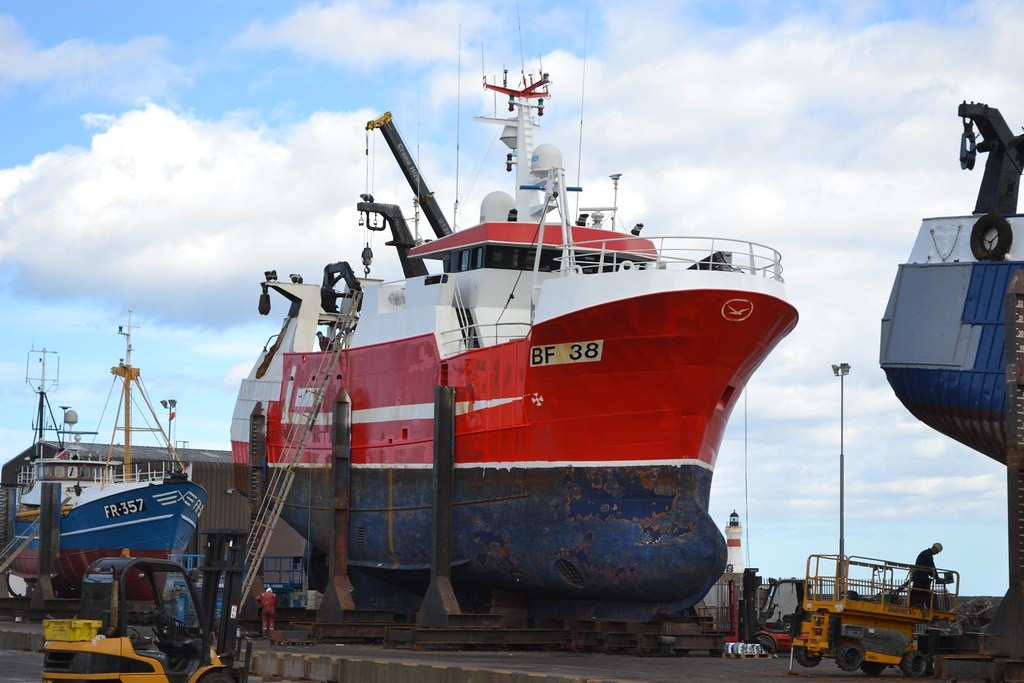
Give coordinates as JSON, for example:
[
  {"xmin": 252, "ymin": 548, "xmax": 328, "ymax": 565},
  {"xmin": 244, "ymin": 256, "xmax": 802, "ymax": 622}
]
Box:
[
  {"xmin": 266, "ymin": 588, "xmax": 272, "ymax": 592},
  {"xmin": 933, "ymin": 543, "xmax": 943, "ymax": 553}
]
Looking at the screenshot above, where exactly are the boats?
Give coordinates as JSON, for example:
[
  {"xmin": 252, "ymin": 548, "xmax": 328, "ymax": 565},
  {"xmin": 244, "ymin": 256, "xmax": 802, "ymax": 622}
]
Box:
[
  {"xmin": 880, "ymin": 99, "xmax": 1024, "ymax": 468},
  {"xmin": 9, "ymin": 309, "xmax": 209, "ymax": 598},
  {"xmin": 230, "ymin": 59, "xmax": 803, "ymax": 629}
]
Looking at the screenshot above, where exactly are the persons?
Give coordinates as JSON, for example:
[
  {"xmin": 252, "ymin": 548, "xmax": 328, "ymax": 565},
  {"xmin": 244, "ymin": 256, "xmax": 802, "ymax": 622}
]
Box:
[
  {"xmin": 255, "ymin": 586, "xmax": 278, "ymax": 643},
  {"xmin": 910, "ymin": 543, "xmax": 942, "ymax": 609},
  {"xmin": 315, "ymin": 331, "xmax": 334, "ymax": 351}
]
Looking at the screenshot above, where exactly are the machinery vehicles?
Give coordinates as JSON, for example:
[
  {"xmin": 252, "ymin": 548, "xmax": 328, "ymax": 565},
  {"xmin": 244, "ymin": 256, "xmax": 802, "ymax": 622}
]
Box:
[
  {"xmin": 740, "ymin": 564, "xmax": 809, "ymax": 658},
  {"xmin": 40, "ymin": 548, "xmax": 255, "ymax": 683},
  {"xmin": 791, "ymin": 554, "xmax": 959, "ymax": 678}
]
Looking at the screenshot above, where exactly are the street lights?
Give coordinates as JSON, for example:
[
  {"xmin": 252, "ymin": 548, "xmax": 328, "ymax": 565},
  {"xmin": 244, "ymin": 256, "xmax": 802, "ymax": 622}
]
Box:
[
  {"xmin": 831, "ymin": 362, "xmax": 850, "ymax": 591},
  {"xmin": 160, "ymin": 399, "xmax": 177, "ymax": 462}
]
